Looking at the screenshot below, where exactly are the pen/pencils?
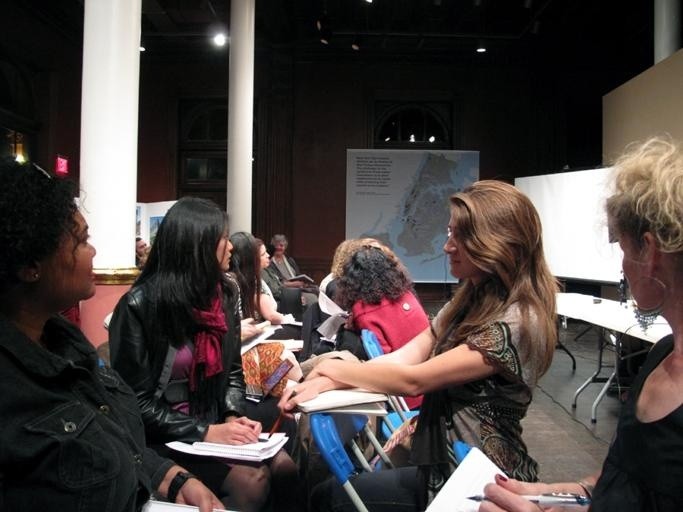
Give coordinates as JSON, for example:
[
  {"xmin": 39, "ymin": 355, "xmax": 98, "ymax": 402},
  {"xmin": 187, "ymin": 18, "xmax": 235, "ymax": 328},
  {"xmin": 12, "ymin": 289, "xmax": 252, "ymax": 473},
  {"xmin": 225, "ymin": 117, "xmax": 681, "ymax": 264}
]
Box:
[
  {"xmin": 268, "ymin": 390, "xmax": 298, "ymax": 439},
  {"xmin": 466, "ymin": 493, "xmax": 592, "ymax": 506},
  {"xmin": 258, "ymin": 438, "xmax": 269, "ymax": 442}
]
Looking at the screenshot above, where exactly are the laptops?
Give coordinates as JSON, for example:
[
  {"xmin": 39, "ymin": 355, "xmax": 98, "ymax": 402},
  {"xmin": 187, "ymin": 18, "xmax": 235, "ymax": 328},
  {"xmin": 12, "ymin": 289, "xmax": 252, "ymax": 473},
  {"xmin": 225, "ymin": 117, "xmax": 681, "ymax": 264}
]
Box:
[{"xmin": 245, "ymin": 357, "xmax": 295, "ymax": 403}]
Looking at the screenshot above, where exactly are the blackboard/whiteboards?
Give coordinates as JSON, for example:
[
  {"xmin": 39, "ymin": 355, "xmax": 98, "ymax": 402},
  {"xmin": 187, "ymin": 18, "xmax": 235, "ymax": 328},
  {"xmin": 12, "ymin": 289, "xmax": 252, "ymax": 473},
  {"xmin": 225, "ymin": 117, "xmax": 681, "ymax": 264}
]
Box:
[{"xmin": 514, "ymin": 165, "xmax": 624, "ymax": 283}]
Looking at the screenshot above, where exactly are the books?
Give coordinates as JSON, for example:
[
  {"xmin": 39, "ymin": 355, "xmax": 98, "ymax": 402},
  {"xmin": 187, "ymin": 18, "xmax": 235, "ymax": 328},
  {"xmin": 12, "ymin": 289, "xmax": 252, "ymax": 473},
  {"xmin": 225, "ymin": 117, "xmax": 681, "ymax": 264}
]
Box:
[{"xmin": 190, "ymin": 429, "xmax": 287, "ymax": 457}]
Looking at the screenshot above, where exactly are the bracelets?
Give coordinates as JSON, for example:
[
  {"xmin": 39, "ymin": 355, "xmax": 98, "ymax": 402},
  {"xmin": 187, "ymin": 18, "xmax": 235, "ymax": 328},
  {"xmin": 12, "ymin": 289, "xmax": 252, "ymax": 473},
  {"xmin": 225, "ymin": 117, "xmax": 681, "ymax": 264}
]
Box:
[{"xmin": 165, "ymin": 470, "xmax": 201, "ymax": 502}]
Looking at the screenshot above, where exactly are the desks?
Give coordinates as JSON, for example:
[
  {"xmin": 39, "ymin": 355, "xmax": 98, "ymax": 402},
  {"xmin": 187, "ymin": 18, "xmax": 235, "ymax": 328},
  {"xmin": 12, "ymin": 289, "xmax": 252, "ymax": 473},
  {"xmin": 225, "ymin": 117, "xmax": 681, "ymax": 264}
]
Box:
[{"xmin": 554, "ymin": 291, "xmax": 673, "ymax": 422}]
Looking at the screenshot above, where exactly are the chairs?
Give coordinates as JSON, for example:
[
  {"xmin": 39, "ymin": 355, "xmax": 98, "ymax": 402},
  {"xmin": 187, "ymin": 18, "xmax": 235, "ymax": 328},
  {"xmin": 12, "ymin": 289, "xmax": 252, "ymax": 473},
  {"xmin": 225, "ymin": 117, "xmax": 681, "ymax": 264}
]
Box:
[
  {"xmin": 361, "ymin": 329, "xmax": 420, "ymax": 445},
  {"xmin": 311, "ymin": 410, "xmax": 395, "ymax": 512}
]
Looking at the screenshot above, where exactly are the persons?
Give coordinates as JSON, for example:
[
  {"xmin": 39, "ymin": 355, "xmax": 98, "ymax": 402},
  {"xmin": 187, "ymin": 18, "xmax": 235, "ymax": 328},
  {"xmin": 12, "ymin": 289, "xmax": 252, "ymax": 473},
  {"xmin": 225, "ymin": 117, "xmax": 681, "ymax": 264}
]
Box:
[
  {"xmin": 103, "ymin": 194, "xmax": 305, "ymax": 510},
  {"xmin": 135, "ymin": 237, "xmax": 152, "ymax": 270},
  {"xmin": 476, "ymin": 126, "xmax": 681, "ymax": 510},
  {"xmin": 0, "ymin": 151, "xmax": 231, "ymax": 512},
  {"xmin": 274, "ymin": 175, "xmax": 562, "ymax": 511},
  {"xmin": 217, "ymin": 236, "xmax": 434, "ymax": 413}
]
